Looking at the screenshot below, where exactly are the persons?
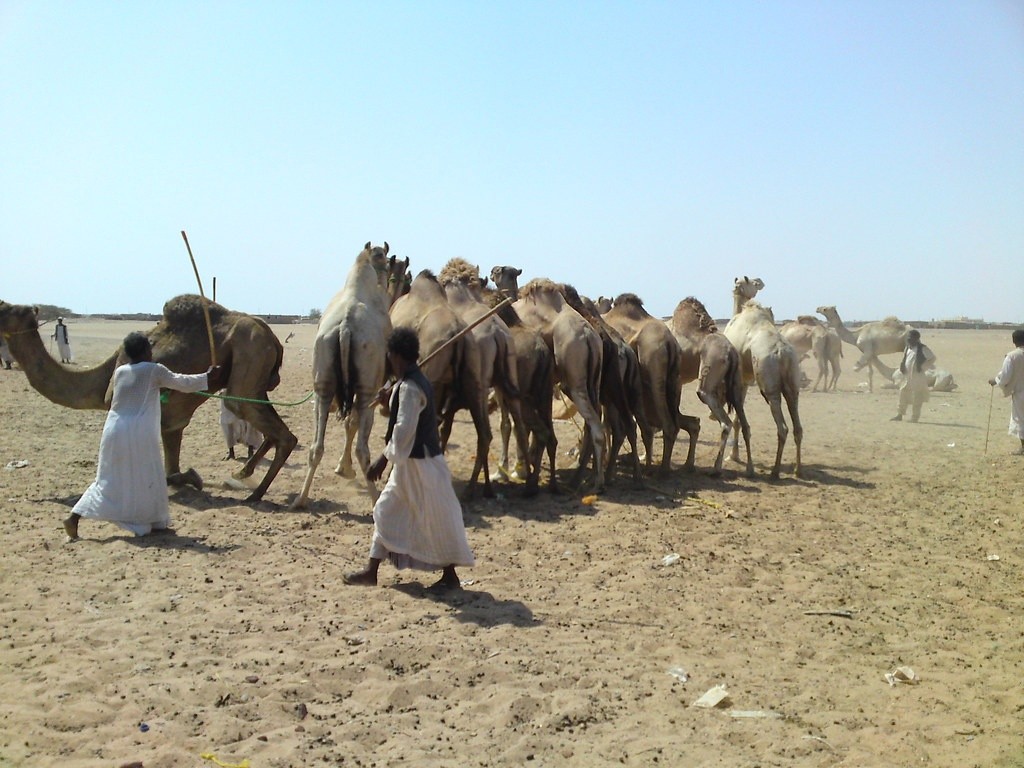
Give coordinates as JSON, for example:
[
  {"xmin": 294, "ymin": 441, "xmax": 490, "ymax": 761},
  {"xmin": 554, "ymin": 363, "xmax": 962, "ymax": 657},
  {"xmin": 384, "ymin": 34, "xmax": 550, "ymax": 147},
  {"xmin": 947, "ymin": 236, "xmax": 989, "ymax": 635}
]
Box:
[
  {"xmin": 988, "ymin": 330, "xmax": 1024, "ymax": 455},
  {"xmin": 218, "ymin": 389, "xmax": 264, "ymax": 460},
  {"xmin": 51, "ymin": 317, "xmax": 71, "ymax": 363},
  {"xmin": 892, "ymin": 329, "xmax": 936, "ymax": 422},
  {"xmin": 341, "ymin": 328, "xmax": 475, "ymax": 593},
  {"xmin": 62, "ymin": 331, "xmax": 222, "ymax": 540},
  {"xmin": 0, "ymin": 338, "xmax": 14, "ymax": 370}
]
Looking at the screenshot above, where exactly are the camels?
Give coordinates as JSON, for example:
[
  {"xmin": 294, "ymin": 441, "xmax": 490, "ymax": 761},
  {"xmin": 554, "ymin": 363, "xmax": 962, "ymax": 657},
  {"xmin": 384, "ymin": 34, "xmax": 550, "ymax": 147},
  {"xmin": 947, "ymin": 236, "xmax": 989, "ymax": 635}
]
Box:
[{"xmin": 0, "ymin": 240, "xmax": 958, "ymax": 508}]
